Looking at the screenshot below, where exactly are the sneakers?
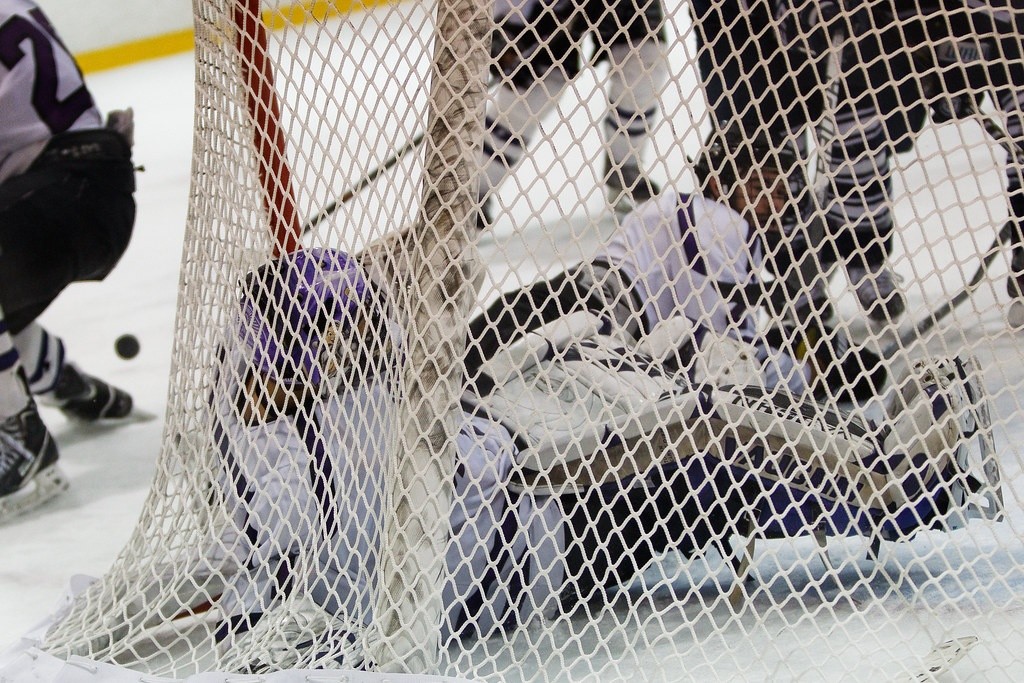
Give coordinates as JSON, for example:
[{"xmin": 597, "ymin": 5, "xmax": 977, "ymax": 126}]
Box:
[
  {"xmin": 845, "ymin": 261, "xmax": 907, "ymax": 321},
  {"xmin": 882, "ymin": 357, "xmax": 1006, "ymax": 539},
  {"xmin": 60, "ymin": 363, "xmax": 152, "ymax": 424},
  {"xmin": 0, "ymin": 400, "xmax": 67, "ymax": 518},
  {"xmin": 602, "ymin": 151, "xmax": 661, "ymax": 218}
]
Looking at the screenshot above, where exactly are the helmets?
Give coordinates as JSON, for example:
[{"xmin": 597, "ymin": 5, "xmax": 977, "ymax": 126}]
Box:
[
  {"xmin": 237, "ymin": 248, "xmax": 396, "ymax": 400},
  {"xmin": 697, "ymin": 120, "xmax": 810, "ymax": 245}
]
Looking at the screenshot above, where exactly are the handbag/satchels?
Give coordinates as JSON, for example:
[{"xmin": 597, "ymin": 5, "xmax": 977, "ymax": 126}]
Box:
[{"xmin": 3, "ymin": 129, "xmax": 136, "ymax": 282}]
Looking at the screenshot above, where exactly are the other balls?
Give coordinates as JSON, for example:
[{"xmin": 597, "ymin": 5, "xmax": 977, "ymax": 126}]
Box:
[{"xmin": 112, "ymin": 332, "xmax": 142, "ymax": 361}]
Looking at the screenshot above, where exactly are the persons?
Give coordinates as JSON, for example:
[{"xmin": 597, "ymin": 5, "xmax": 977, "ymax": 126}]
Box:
[
  {"xmin": 476, "ymin": 1, "xmax": 667, "ymax": 232},
  {"xmin": 1, "ymin": 1, "xmax": 137, "ymax": 522},
  {"xmin": 606, "ymin": 119, "xmax": 888, "ymax": 402},
  {"xmin": 206, "ymin": 249, "xmax": 1005, "ymax": 674},
  {"xmin": 688, "ymin": 0, "xmax": 1024, "ymax": 322}
]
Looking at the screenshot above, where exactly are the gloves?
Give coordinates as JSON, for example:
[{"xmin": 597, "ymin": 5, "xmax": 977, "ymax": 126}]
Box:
[
  {"xmin": 358, "ymin": 226, "xmax": 486, "ymax": 321},
  {"xmin": 922, "ymin": 37, "xmax": 989, "ymax": 124}
]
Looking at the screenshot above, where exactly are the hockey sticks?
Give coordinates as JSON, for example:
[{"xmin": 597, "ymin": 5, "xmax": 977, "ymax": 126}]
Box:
[{"xmin": 234, "ymin": 129, "xmax": 426, "ymax": 274}]
